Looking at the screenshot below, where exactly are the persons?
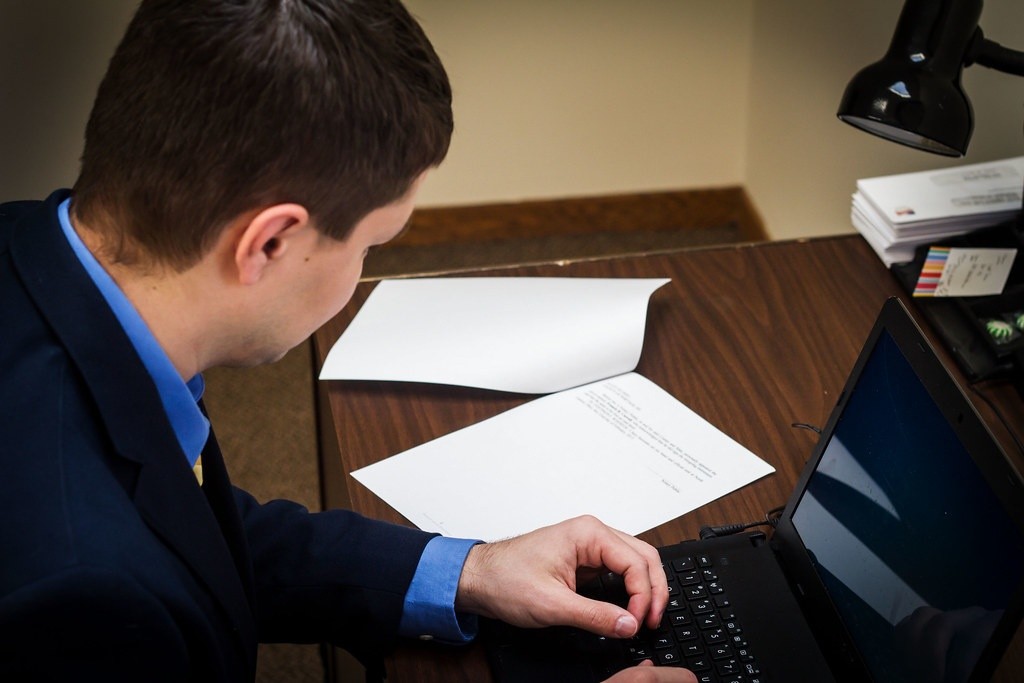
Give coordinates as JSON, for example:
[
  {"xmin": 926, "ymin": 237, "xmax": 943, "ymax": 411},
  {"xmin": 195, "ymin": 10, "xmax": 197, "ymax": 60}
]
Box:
[{"xmin": 0, "ymin": 0, "xmax": 698, "ymax": 683}]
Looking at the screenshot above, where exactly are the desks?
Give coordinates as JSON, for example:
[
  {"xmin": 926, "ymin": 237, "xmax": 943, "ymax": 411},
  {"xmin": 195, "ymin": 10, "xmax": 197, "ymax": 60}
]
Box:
[{"xmin": 309, "ymin": 231, "xmax": 1024, "ymax": 683}]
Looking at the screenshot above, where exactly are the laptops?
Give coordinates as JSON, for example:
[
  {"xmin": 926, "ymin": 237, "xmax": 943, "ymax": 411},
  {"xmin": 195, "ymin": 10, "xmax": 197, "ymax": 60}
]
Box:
[{"xmin": 481, "ymin": 295, "xmax": 1024, "ymax": 683}]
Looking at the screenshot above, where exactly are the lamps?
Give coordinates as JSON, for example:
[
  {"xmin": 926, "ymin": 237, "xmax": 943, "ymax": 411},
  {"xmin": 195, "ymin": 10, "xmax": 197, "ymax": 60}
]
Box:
[{"xmin": 836, "ymin": 0, "xmax": 1024, "ymax": 159}]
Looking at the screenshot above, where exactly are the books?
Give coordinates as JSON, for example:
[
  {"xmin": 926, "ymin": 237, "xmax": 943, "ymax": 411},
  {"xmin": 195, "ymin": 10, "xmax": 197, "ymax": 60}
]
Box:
[{"xmin": 851, "ymin": 157, "xmax": 1024, "ymax": 270}]
